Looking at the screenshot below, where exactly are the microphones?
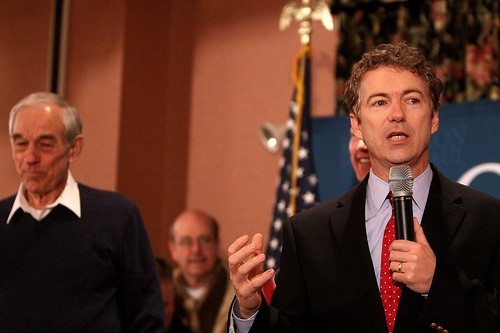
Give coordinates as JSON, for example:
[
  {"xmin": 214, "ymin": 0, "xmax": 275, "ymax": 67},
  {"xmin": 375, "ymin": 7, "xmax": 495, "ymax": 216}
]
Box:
[{"xmin": 388, "ymin": 165, "xmax": 414, "ymax": 242}]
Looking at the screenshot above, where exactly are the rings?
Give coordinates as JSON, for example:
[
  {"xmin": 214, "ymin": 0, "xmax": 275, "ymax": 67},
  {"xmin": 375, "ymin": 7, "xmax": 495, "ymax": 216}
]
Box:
[{"xmin": 399, "ymin": 262, "xmax": 402, "ymax": 272}]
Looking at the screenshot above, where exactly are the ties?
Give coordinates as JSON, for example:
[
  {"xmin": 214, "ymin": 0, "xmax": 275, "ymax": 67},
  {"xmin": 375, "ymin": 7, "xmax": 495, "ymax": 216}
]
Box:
[{"xmin": 379, "ymin": 191, "xmax": 406, "ymax": 332}]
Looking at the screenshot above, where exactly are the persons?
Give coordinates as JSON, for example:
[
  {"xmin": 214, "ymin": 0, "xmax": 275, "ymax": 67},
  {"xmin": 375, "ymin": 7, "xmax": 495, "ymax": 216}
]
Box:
[
  {"xmin": 0, "ymin": 91, "xmax": 168, "ymax": 333},
  {"xmin": 348, "ymin": 129, "xmax": 372, "ymax": 182},
  {"xmin": 167, "ymin": 210, "xmax": 239, "ymax": 333},
  {"xmin": 228, "ymin": 43, "xmax": 500, "ymax": 333}
]
